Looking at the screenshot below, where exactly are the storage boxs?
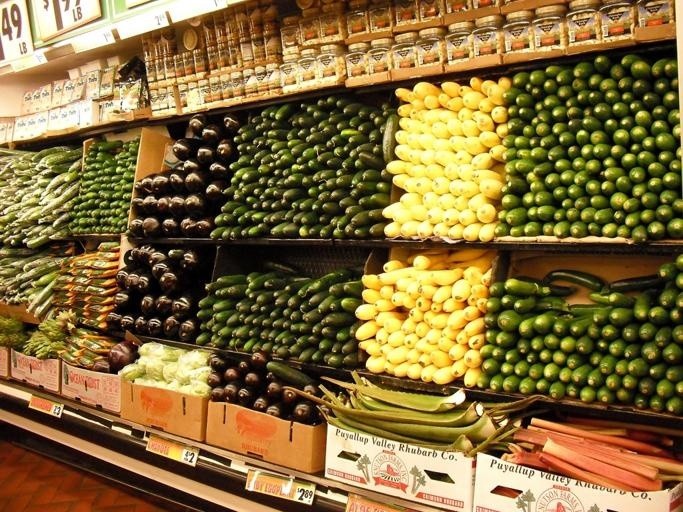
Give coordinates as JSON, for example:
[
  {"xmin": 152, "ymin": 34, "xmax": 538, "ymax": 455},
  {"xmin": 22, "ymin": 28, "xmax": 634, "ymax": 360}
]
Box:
[
  {"xmin": 323, "ymin": 422, "xmax": 476, "ymax": 512},
  {"xmin": 9, "ymin": 347, "xmax": 61, "ymax": 394},
  {"xmin": 473, "ymin": 450, "xmax": 683, "ymax": 512},
  {"xmin": 1, "ymin": 346, "xmax": 9, "ymax": 378},
  {"xmin": 205, "ymin": 400, "xmax": 326, "ymax": 474},
  {"xmin": 120, "ymin": 369, "xmax": 212, "ymax": 443},
  {"xmin": 61, "ymin": 361, "xmax": 121, "ymax": 414}
]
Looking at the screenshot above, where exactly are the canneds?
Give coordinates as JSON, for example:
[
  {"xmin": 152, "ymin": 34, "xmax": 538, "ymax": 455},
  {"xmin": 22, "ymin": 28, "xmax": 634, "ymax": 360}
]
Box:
[{"xmin": 142, "ymin": 0, "xmax": 675, "ymax": 117}]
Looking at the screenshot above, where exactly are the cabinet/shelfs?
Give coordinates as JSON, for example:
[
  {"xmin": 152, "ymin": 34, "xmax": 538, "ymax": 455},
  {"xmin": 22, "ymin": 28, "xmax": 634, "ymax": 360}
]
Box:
[{"xmin": 0, "ymin": 0, "xmax": 682, "ymax": 512}]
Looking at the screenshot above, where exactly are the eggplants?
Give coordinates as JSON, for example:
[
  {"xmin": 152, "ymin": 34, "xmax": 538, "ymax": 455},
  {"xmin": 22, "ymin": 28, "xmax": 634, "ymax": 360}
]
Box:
[{"xmin": 68, "ymin": 53, "xmax": 683, "ymax": 427}]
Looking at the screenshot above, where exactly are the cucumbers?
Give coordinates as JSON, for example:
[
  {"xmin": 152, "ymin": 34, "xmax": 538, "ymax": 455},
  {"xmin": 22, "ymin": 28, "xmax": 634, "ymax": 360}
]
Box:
[{"xmin": 0, "ymin": 145, "xmax": 83, "ymax": 319}]
